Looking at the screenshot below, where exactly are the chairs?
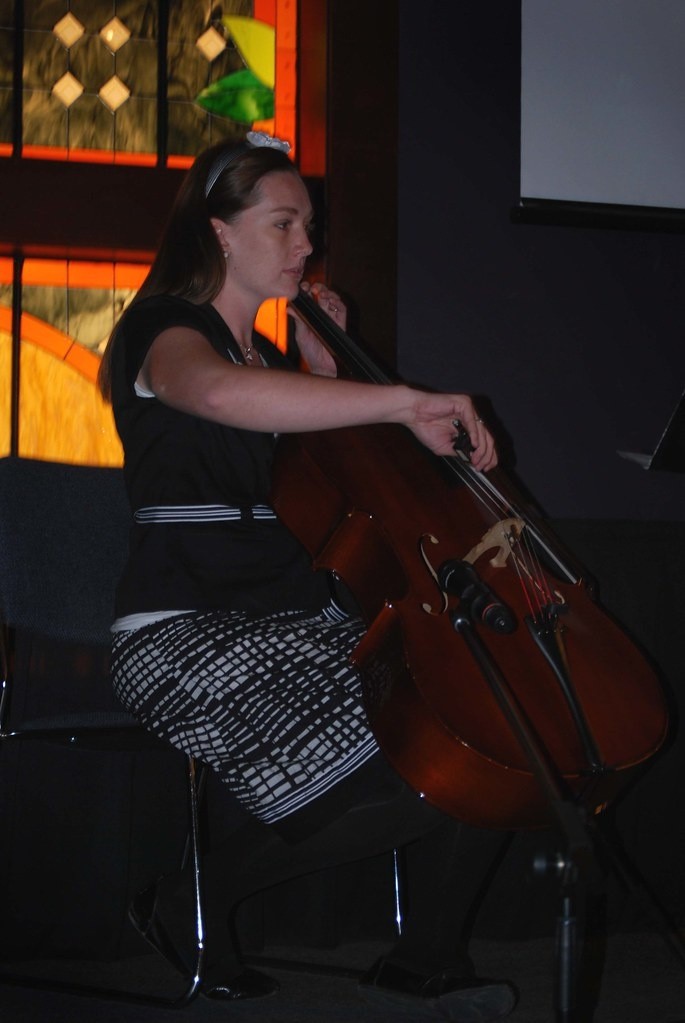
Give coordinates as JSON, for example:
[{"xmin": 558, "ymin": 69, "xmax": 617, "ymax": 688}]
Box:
[{"xmin": 0, "ymin": 457, "xmax": 406, "ymax": 1022}]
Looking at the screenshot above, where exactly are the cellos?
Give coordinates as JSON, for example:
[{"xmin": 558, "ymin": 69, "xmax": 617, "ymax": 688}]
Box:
[{"xmin": 261, "ymin": 288, "xmax": 684, "ymax": 965}]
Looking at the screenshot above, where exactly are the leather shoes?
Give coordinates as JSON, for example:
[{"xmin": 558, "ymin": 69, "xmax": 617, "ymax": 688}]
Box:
[
  {"xmin": 358, "ymin": 955, "xmax": 520, "ymax": 1022},
  {"xmin": 129, "ymin": 887, "xmax": 279, "ymax": 1000}
]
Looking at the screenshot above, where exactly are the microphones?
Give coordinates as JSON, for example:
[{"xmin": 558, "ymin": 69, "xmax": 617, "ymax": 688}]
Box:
[{"xmin": 437, "ymin": 557, "xmax": 516, "ymax": 634}]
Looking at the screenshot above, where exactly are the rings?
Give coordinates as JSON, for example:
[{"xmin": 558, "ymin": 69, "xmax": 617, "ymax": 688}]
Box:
[{"xmin": 473, "ymin": 418, "xmax": 486, "ymax": 423}]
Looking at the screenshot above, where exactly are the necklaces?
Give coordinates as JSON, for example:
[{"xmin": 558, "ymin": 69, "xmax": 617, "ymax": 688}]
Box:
[{"xmin": 239, "ymin": 343, "xmax": 255, "ymax": 360}]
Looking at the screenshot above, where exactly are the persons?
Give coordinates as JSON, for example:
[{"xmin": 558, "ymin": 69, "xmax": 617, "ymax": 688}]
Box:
[{"xmin": 96, "ymin": 131, "xmax": 519, "ymax": 1023}]
